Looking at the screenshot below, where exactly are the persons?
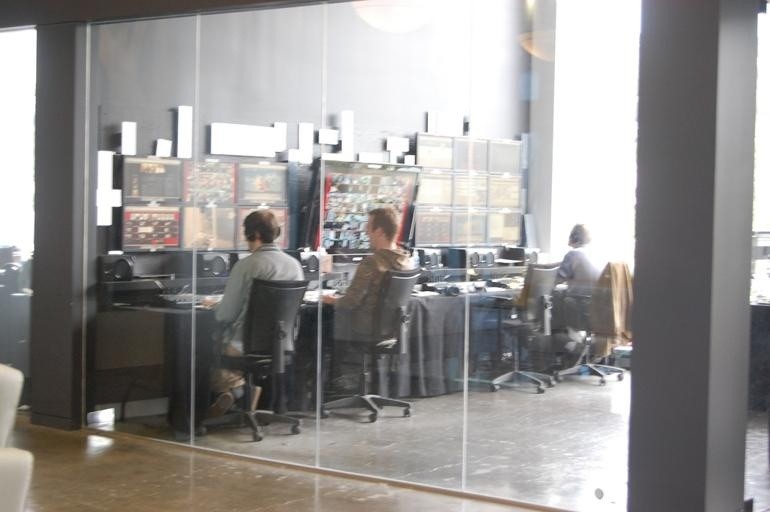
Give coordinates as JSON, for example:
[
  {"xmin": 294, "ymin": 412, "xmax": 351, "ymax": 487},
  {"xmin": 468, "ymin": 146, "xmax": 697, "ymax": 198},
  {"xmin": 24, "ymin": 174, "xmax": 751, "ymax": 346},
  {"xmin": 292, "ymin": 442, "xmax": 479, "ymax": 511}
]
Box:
[
  {"xmin": 295, "ymin": 209, "xmax": 418, "ymax": 356},
  {"xmin": 207, "ymin": 211, "xmax": 305, "ymax": 417},
  {"xmin": 551, "ymin": 224, "xmax": 600, "ymax": 334}
]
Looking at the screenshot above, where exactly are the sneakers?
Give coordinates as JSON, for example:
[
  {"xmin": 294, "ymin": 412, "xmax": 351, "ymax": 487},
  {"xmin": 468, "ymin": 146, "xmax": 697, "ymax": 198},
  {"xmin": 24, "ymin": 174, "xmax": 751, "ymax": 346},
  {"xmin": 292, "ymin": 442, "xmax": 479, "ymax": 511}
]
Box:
[{"xmin": 207, "ymin": 392, "xmax": 235, "ymax": 421}]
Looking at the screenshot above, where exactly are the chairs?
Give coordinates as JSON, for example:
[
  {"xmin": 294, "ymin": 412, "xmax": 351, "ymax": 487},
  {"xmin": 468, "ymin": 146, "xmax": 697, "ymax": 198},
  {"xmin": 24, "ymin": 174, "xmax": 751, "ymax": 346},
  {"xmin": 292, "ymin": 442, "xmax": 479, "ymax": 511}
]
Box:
[
  {"xmin": 321, "ymin": 268, "xmax": 422, "ymax": 422},
  {"xmin": 470, "ymin": 259, "xmax": 559, "ymax": 394},
  {"xmin": 555, "ymin": 261, "xmax": 633, "ymax": 386},
  {"xmin": 202, "ymin": 277, "xmax": 309, "ymax": 437}
]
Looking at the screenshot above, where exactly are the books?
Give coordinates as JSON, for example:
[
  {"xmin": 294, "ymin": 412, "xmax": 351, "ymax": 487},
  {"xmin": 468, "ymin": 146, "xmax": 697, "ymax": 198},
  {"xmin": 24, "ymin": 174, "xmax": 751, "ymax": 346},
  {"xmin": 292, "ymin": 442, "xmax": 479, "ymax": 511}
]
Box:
[{"xmin": 303, "ymin": 289, "xmax": 339, "ymax": 302}]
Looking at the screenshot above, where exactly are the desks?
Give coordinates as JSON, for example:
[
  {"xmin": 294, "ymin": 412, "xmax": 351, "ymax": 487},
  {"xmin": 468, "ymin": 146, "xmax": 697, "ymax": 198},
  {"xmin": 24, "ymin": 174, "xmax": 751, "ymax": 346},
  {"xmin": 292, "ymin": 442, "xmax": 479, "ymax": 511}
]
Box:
[{"xmin": 95, "ymin": 276, "xmax": 562, "ymax": 439}]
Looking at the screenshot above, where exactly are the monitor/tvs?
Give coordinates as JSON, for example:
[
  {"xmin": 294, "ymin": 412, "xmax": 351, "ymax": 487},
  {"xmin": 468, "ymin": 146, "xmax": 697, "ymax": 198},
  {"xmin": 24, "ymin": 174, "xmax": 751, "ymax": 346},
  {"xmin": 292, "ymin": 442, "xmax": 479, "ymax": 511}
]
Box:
[
  {"xmin": 301, "ymin": 158, "xmax": 423, "ymax": 253},
  {"xmin": 413, "ymin": 130, "xmax": 524, "ymax": 249},
  {"xmin": 119, "ymin": 154, "xmax": 291, "ymax": 253}
]
